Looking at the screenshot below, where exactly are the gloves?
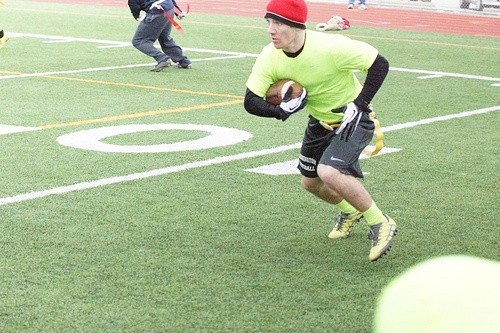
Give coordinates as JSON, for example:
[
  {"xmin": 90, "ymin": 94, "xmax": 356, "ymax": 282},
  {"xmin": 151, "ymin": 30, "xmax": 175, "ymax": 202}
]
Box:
[
  {"xmin": 274, "ymin": 87, "xmax": 307, "ymax": 122},
  {"xmin": 331, "ymin": 99, "xmax": 372, "ymax": 142}
]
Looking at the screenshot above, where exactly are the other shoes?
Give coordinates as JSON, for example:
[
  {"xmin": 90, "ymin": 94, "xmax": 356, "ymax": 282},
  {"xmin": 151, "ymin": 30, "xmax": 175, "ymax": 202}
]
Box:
[
  {"xmin": 348, "ymin": 3, "xmax": 354, "ymax": 8},
  {"xmin": 358, "ymin": 3, "xmax": 367, "ymax": 9}
]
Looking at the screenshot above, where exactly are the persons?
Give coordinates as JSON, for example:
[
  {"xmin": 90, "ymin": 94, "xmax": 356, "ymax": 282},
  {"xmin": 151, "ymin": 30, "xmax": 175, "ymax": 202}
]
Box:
[
  {"xmin": 244, "ymin": 0, "xmax": 397, "ymax": 261},
  {"xmin": 128, "ymin": 0, "xmax": 193, "ymax": 72},
  {"xmin": 348, "ymin": 0, "xmax": 368, "ymax": 10}
]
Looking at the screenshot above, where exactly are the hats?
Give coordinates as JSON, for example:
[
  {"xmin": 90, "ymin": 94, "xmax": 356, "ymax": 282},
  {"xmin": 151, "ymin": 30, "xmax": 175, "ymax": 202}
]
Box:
[{"xmin": 264, "ymin": 0, "xmax": 308, "ymax": 29}]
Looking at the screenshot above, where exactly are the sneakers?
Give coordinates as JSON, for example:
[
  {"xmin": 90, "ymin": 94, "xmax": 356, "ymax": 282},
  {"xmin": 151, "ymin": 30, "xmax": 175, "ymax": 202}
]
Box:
[
  {"xmin": 150, "ymin": 58, "xmax": 173, "ymax": 72},
  {"xmin": 328, "ymin": 211, "xmax": 364, "ymax": 239},
  {"xmin": 368, "ymin": 213, "xmax": 397, "ymax": 262},
  {"xmin": 176, "ymin": 62, "xmax": 192, "ymax": 69}
]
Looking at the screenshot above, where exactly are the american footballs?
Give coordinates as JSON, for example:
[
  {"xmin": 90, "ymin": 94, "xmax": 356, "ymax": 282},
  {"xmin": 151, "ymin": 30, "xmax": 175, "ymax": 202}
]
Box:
[{"xmin": 266, "ymin": 78, "xmax": 307, "ymax": 114}]
